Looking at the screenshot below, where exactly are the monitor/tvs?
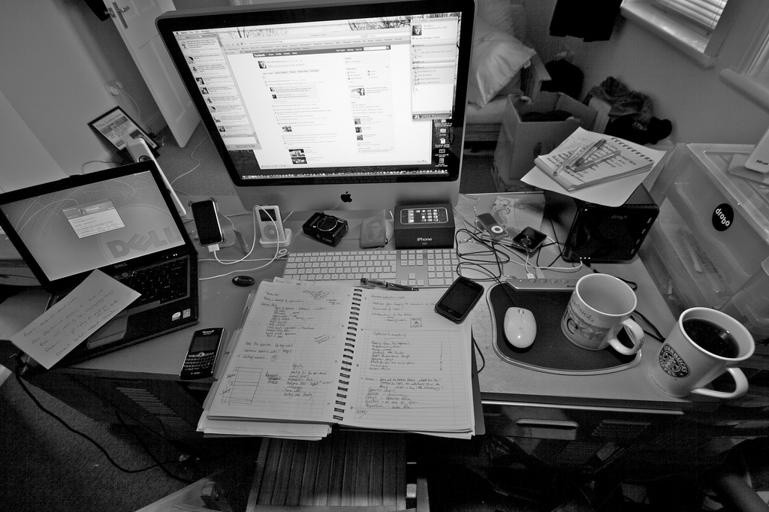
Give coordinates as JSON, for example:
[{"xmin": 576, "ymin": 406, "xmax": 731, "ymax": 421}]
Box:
[{"xmin": 153, "ymin": 0, "xmax": 475, "ymax": 216}]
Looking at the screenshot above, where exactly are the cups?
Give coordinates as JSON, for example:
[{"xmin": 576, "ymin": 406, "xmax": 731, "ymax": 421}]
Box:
[
  {"xmin": 561, "ymin": 273, "xmax": 645, "ymax": 356},
  {"xmin": 649, "ymin": 306, "xmax": 756, "ymax": 400}
]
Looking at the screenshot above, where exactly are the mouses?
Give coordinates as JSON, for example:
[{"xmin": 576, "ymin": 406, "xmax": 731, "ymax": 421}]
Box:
[{"xmin": 504, "ymin": 304, "xmax": 538, "ymax": 352}]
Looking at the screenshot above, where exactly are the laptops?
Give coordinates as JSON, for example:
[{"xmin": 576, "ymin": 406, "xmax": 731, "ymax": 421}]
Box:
[{"xmin": 0, "ymin": 158, "xmax": 201, "ymax": 370}]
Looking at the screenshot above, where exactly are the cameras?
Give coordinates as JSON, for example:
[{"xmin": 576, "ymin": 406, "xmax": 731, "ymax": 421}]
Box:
[{"xmin": 301, "ymin": 208, "xmax": 349, "ymax": 248}]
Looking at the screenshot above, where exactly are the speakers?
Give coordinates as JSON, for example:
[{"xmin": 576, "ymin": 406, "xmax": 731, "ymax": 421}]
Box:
[
  {"xmin": 130, "ymin": 135, "xmax": 188, "ymax": 225},
  {"xmin": 540, "ymin": 169, "xmax": 661, "ymax": 262}
]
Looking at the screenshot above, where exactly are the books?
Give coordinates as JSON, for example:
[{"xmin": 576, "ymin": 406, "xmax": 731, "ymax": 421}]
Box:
[
  {"xmin": 198, "ymin": 276, "xmax": 476, "ymax": 444},
  {"xmin": 532, "ymin": 136, "xmax": 655, "ymax": 193}
]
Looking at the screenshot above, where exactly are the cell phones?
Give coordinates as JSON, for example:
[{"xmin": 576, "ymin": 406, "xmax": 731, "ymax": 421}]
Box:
[
  {"xmin": 175, "ymin": 326, "xmax": 227, "ymax": 383},
  {"xmin": 434, "ymin": 275, "xmax": 485, "ymax": 323},
  {"xmin": 511, "ymin": 226, "xmax": 548, "ymax": 258},
  {"xmin": 189, "ymin": 201, "xmax": 226, "ymax": 247}
]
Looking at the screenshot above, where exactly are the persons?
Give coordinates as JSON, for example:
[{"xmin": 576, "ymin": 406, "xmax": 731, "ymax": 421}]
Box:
[
  {"xmin": 412, "ymin": 25, "xmax": 422, "ymax": 36},
  {"xmin": 258, "ymin": 60, "xmax": 267, "ymax": 70}
]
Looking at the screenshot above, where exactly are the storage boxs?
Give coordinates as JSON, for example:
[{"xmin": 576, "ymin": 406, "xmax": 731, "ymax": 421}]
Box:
[{"xmin": 639, "ymin": 142, "xmax": 769, "ymax": 352}]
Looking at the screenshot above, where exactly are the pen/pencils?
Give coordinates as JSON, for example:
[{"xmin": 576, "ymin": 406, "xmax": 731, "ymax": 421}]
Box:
[
  {"xmin": 552, "ymin": 138, "xmax": 621, "ymax": 176},
  {"xmin": 360, "ymin": 277, "xmax": 419, "ymax": 292}
]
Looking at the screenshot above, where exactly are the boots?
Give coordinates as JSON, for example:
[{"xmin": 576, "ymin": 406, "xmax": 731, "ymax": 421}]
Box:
[{"xmin": 582, "ymin": 77, "xmax": 672, "ymax": 145}]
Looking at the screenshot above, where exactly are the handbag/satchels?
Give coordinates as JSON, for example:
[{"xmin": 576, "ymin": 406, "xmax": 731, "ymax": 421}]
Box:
[{"xmin": 489, "ymin": 91, "xmax": 598, "ymax": 192}]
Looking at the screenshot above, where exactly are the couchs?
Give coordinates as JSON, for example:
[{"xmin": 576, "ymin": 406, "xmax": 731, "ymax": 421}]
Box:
[{"xmin": 465, "ymin": 0, "xmax": 552, "ymax": 143}]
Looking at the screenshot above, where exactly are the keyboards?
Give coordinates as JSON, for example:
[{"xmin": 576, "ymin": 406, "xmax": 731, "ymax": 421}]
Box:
[{"xmin": 281, "ymin": 250, "xmax": 462, "ymax": 289}]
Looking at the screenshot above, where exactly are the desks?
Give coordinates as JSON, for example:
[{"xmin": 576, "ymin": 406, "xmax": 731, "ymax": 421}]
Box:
[{"xmin": 0, "ymin": 189, "xmax": 697, "ymax": 480}]
